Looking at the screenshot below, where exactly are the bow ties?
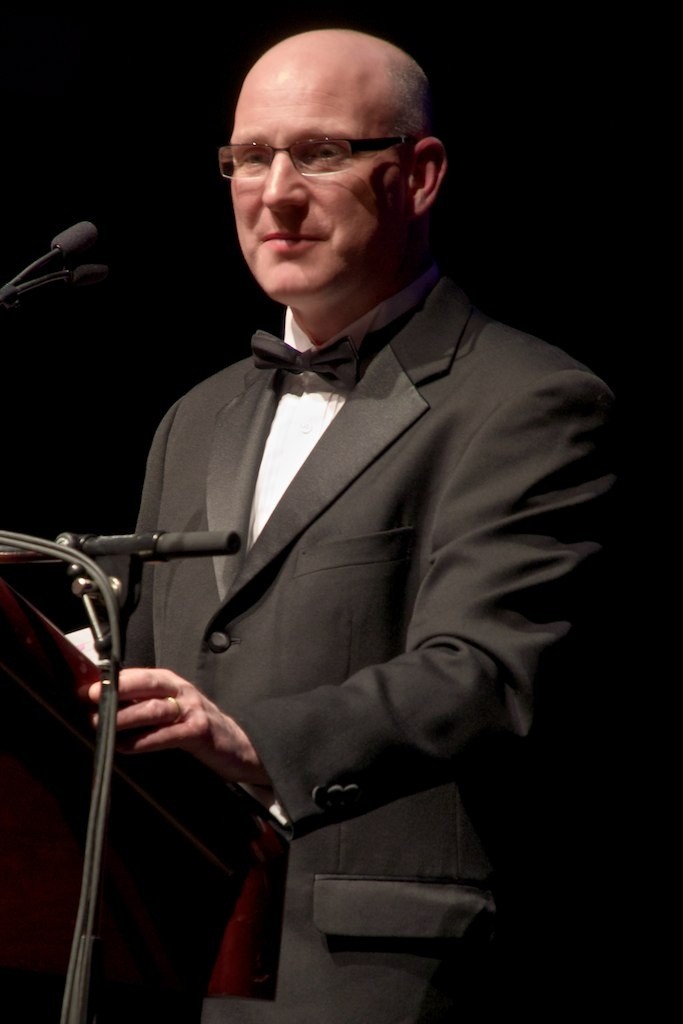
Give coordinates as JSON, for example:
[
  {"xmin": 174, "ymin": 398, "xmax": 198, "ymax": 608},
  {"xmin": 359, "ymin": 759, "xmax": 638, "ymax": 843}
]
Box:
[{"xmin": 250, "ymin": 307, "xmax": 418, "ymax": 389}]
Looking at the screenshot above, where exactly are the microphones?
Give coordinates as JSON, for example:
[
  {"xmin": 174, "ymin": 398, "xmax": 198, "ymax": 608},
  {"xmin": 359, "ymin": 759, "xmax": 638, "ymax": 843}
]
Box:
[{"xmin": 1, "ymin": 220, "xmax": 108, "ymax": 304}]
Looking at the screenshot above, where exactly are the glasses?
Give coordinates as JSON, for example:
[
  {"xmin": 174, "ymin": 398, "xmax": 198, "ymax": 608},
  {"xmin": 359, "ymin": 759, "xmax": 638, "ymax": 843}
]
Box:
[{"xmin": 217, "ymin": 136, "xmax": 420, "ymax": 180}]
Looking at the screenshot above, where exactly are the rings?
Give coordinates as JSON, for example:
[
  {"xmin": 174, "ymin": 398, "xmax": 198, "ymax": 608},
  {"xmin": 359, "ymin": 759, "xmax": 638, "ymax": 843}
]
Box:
[{"xmin": 166, "ymin": 696, "xmax": 181, "ymax": 723}]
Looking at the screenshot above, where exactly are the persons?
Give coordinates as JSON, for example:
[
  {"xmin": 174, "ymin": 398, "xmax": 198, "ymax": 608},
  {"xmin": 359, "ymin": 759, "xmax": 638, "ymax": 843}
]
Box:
[{"xmin": 88, "ymin": 29, "xmax": 624, "ymax": 1024}]
